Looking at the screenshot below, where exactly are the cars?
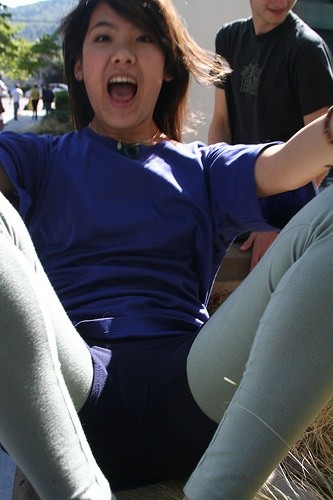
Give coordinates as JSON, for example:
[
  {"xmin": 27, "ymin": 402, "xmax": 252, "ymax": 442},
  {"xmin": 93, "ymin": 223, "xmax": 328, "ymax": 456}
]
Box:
[{"xmin": 25, "ymin": 82, "xmax": 69, "ymax": 97}]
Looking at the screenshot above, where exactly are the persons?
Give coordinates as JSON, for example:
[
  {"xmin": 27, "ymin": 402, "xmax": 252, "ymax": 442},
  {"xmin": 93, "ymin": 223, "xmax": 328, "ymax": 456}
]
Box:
[
  {"xmin": 10, "ymin": 83, "xmax": 23, "ymax": 120},
  {"xmin": 209, "ymin": 0, "xmax": 333, "ymax": 275},
  {"xmin": 0, "ymin": 75, "xmax": 7, "ymax": 131},
  {"xmin": 31, "ymin": 85, "xmax": 39, "ymax": 116},
  {"xmin": 42, "ymin": 85, "xmax": 54, "ymax": 114},
  {"xmin": 0, "ymin": 0, "xmax": 333, "ymax": 500}
]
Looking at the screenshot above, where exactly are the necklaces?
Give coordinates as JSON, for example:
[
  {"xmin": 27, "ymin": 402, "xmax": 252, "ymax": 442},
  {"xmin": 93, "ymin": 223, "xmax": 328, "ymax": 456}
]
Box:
[{"xmin": 89, "ymin": 123, "xmax": 160, "ymax": 149}]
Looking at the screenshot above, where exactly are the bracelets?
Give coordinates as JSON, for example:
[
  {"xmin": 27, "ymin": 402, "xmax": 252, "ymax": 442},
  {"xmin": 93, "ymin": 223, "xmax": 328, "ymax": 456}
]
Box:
[{"xmin": 324, "ymin": 106, "xmax": 333, "ymax": 144}]
